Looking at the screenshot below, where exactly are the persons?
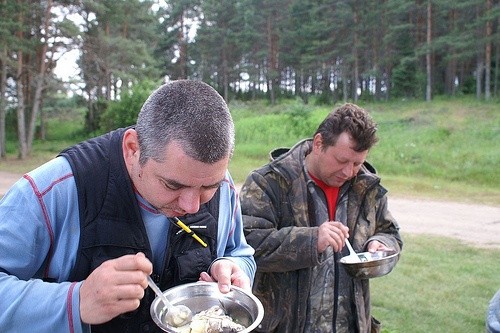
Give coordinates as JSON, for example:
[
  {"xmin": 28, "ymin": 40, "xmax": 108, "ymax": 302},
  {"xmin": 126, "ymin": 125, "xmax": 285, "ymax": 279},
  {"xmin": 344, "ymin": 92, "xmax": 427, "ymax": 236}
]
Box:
[
  {"xmin": 0, "ymin": 79, "xmax": 257, "ymax": 333},
  {"xmin": 240, "ymin": 102, "xmax": 403, "ymax": 332}
]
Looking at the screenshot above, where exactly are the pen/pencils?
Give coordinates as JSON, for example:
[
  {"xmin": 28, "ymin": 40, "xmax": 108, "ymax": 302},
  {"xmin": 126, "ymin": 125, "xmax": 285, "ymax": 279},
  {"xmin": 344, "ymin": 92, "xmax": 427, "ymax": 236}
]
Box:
[{"xmin": 174, "ymin": 217, "xmax": 207, "ymax": 247}]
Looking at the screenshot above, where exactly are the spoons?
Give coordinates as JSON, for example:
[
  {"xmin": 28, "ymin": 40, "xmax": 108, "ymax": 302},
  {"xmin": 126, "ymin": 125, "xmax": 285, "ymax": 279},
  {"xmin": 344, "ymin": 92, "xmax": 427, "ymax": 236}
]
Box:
[
  {"xmin": 343, "ymin": 237, "xmax": 362, "ymax": 263},
  {"xmin": 146, "ymin": 273, "xmax": 193, "ymax": 326}
]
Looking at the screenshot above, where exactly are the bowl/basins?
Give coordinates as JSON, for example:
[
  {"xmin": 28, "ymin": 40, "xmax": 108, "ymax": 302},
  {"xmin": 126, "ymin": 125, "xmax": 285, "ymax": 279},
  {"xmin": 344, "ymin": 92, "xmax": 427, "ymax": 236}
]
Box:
[
  {"xmin": 150, "ymin": 281, "xmax": 264, "ymax": 333},
  {"xmin": 337, "ymin": 250, "xmax": 400, "ymax": 278}
]
